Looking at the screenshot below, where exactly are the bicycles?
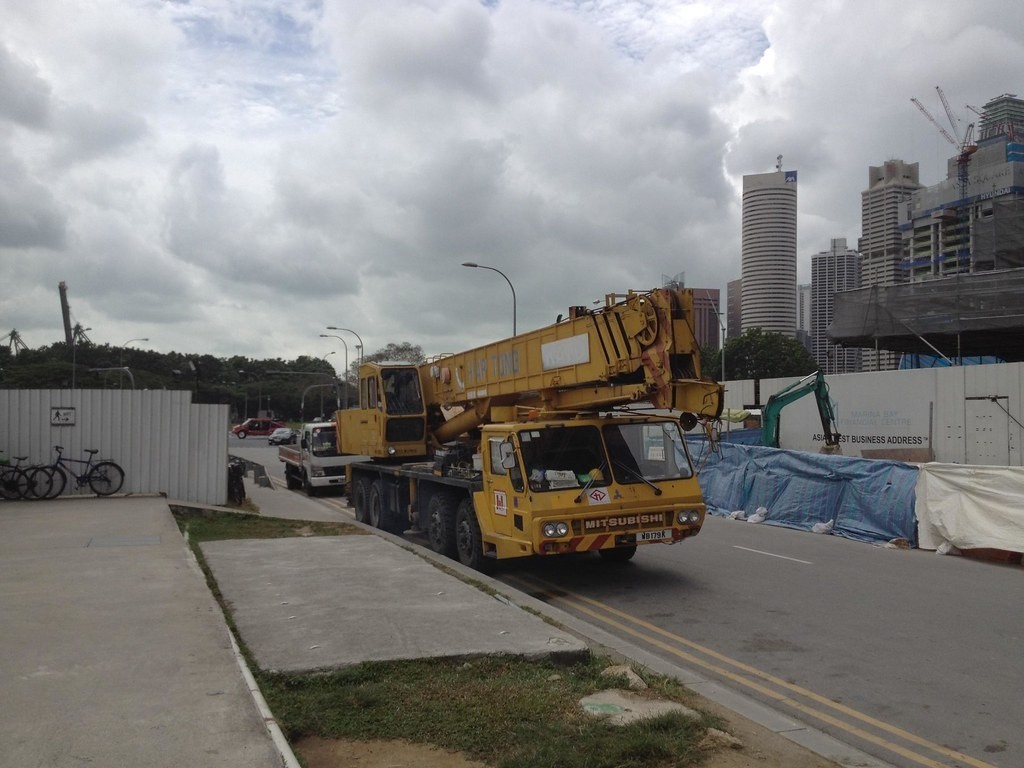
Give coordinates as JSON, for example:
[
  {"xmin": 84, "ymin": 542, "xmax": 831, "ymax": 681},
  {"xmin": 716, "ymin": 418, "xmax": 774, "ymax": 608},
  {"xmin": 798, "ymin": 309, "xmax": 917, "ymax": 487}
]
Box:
[{"xmin": 0, "ymin": 445, "xmax": 125, "ymax": 500}]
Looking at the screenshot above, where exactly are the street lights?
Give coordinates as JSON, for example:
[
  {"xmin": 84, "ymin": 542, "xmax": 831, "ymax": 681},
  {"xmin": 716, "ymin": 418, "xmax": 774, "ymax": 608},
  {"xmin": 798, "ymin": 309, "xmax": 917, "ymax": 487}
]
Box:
[
  {"xmin": 320, "ymin": 335, "xmax": 348, "ymax": 410},
  {"xmin": 462, "ymin": 262, "xmax": 516, "ymax": 336},
  {"xmin": 265, "ymin": 370, "xmax": 340, "ymax": 411},
  {"xmin": 72, "ymin": 327, "xmax": 92, "ymax": 389},
  {"xmin": 120, "ymin": 339, "xmax": 149, "ymax": 389},
  {"xmin": 321, "ymin": 352, "xmax": 335, "ymax": 423},
  {"xmin": 326, "ymin": 327, "xmax": 363, "ymax": 364},
  {"xmin": 240, "ymin": 370, "xmax": 261, "ymax": 418}
]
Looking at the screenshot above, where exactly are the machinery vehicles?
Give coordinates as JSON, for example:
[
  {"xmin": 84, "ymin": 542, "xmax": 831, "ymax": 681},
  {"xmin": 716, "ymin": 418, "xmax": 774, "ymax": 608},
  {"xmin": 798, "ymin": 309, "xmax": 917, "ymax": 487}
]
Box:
[
  {"xmin": 761, "ymin": 369, "xmax": 843, "ymax": 455},
  {"xmin": 334, "ymin": 288, "xmax": 729, "ymax": 577}
]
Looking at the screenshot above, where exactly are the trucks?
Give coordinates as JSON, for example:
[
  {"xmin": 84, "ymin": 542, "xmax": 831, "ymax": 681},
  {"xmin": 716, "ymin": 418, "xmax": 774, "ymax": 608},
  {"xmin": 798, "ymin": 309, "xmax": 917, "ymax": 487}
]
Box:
[{"xmin": 278, "ymin": 422, "xmax": 373, "ymax": 496}]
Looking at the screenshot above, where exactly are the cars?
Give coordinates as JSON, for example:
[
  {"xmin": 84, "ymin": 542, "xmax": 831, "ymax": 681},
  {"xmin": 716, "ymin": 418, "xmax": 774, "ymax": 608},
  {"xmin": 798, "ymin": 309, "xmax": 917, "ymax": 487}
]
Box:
[
  {"xmin": 268, "ymin": 428, "xmax": 297, "ymax": 445},
  {"xmin": 232, "ymin": 418, "xmax": 287, "ymax": 439}
]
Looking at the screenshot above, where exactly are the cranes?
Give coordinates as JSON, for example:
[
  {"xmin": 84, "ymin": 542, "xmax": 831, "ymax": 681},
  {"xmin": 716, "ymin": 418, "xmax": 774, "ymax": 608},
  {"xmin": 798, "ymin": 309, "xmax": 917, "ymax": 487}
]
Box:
[
  {"xmin": 0, "ymin": 281, "xmax": 93, "ymax": 356},
  {"xmin": 910, "ymin": 85, "xmax": 1014, "ymax": 153}
]
